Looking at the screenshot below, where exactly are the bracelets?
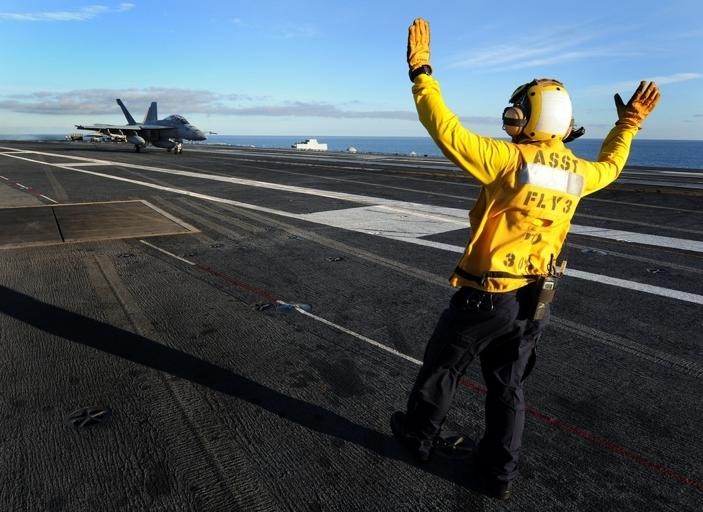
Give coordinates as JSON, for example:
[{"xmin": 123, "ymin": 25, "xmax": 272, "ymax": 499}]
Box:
[{"xmin": 409, "ymin": 65, "xmax": 432, "ymax": 83}]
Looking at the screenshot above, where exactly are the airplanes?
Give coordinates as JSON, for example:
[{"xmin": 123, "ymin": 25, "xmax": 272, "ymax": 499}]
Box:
[{"xmin": 74, "ymin": 99, "xmax": 207, "ymax": 154}]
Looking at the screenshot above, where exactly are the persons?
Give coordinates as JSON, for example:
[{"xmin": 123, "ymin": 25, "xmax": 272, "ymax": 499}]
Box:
[{"xmin": 388, "ymin": 19, "xmax": 662, "ymax": 500}]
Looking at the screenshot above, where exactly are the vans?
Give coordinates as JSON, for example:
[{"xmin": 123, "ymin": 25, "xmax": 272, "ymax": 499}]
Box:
[{"xmin": 83, "ymin": 133, "xmax": 102, "ymax": 144}]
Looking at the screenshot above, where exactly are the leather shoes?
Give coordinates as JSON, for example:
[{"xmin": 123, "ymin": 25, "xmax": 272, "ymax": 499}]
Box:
[
  {"xmin": 462, "ymin": 451, "xmax": 511, "ymax": 500},
  {"xmin": 389, "ymin": 411, "xmax": 441, "ymax": 463}
]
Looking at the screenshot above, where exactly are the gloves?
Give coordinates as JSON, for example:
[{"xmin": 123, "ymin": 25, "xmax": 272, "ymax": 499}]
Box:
[
  {"xmin": 613, "ymin": 79, "xmax": 659, "ymax": 128},
  {"xmin": 407, "ymin": 19, "xmax": 431, "ymax": 64}
]
